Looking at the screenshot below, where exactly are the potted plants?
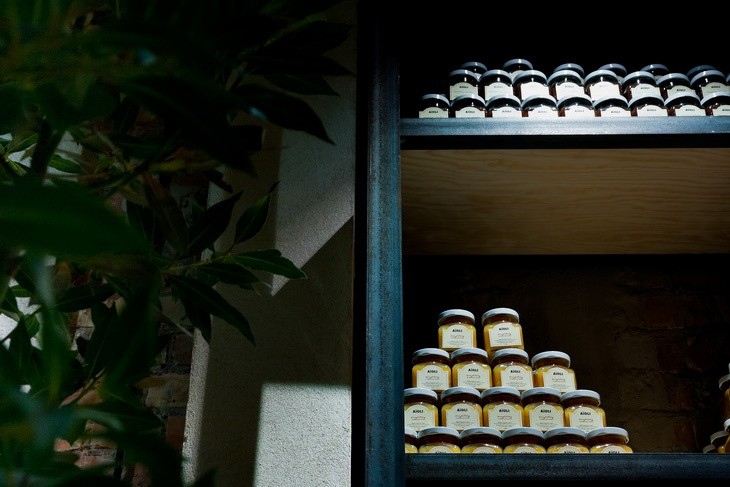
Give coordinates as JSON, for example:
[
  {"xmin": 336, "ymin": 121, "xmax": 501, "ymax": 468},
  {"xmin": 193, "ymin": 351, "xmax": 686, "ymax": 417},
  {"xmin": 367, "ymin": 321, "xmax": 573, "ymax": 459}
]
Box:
[{"xmin": 1, "ymin": 0, "xmax": 350, "ymax": 487}]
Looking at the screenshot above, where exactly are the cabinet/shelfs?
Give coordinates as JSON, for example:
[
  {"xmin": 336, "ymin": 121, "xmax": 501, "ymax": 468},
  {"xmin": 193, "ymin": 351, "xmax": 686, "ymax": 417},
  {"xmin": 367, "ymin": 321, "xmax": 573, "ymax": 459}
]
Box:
[{"xmin": 355, "ymin": 0, "xmax": 730, "ymax": 487}]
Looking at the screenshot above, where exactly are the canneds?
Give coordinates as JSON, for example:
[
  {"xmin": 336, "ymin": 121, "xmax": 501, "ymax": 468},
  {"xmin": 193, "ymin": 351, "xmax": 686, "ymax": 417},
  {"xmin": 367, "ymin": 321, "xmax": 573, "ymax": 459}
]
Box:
[
  {"xmin": 701, "ymin": 364, "xmax": 730, "ymax": 454},
  {"xmin": 403, "ymin": 308, "xmax": 633, "ymax": 454},
  {"xmin": 420, "ymin": 58, "xmax": 730, "ymax": 117}
]
